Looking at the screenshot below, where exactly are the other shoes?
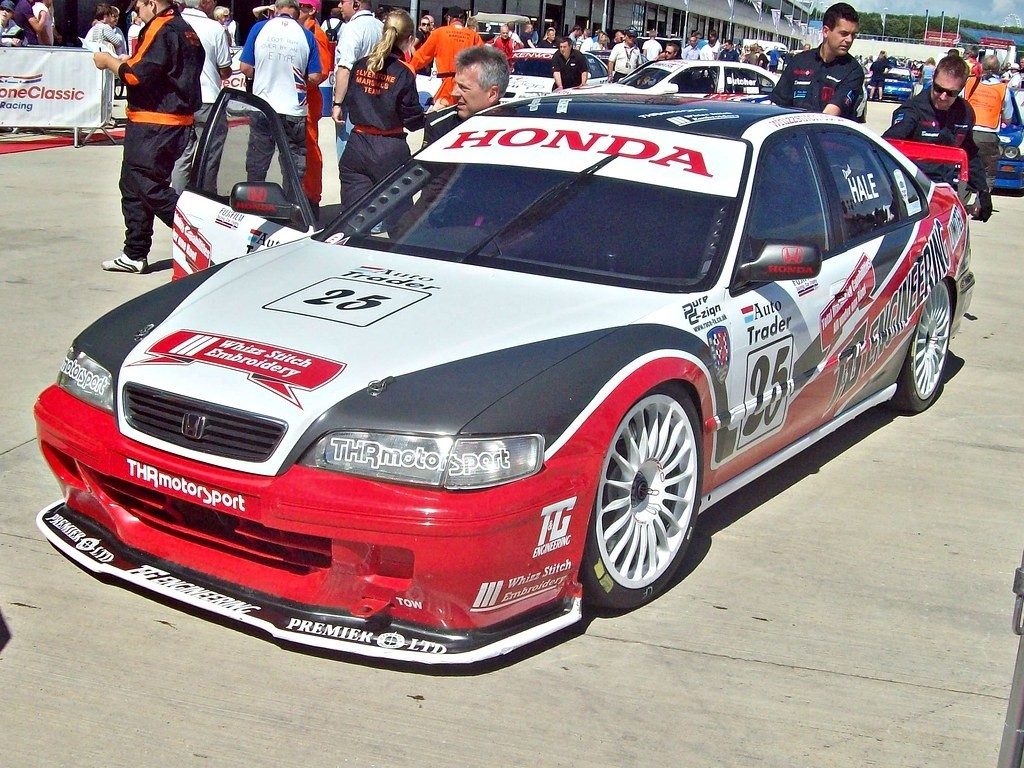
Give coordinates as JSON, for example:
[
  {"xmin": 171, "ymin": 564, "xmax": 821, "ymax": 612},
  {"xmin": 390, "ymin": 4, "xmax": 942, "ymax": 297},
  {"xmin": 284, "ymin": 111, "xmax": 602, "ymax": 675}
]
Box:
[{"xmin": 869, "ymin": 98, "xmax": 882, "ymax": 102}]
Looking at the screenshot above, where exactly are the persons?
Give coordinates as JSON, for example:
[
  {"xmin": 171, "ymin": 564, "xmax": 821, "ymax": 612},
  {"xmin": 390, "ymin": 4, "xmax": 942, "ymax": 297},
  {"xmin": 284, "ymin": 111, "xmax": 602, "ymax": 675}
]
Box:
[
  {"xmin": 767, "ymin": 3, "xmax": 865, "ymax": 116},
  {"xmin": 93, "ymin": 1, "xmax": 205, "ymax": 273},
  {"xmin": 960, "ymin": 57, "xmax": 1013, "ymax": 218},
  {"xmin": 0, "ymin": 1, "xmax": 1023, "ymax": 103},
  {"xmin": 288, "ymin": 0, "xmax": 331, "ymax": 222},
  {"xmin": 340, "ymin": 11, "xmax": 425, "ymax": 248},
  {"xmin": 333, "ymin": 1, "xmax": 391, "ymax": 234},
  {"xmin": 880, "ymin": 55, "xmax": 993, "ymax": 223},
  {"xmin": 239, "ymin": 0, "xmax": 321, "ymax": 213},
  {"xmin": 411, "ymin": 8, "xmax": 486, "ymax": 114},
  {"xmin": 168, "ymin": 0, "xmax": 233, "ymax": 200},
  {"xmin": 422, "ymin": 45, "xmax": 509, "ymax": 163}
]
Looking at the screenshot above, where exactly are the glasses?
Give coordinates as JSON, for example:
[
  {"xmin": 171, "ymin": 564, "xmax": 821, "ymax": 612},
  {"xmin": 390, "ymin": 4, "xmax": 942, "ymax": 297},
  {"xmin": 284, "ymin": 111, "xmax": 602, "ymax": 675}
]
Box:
[
  {"xmin": 933, "ymin": 78, "xmax": 963, "ymax": 98},
  {"xmin": 421, "ymin": 22, "xmax": 430, "ymax": 27},
  {"xmin": 135, "ymin": 0, "xmax": 150, "ymax": 14},
  {"xmin": 665, "ymin": 51, "xmax": 675, "ymax": 54},
  {"xmin": 1, "ymin": 7, "xmax": 10, "ymax": 12}
]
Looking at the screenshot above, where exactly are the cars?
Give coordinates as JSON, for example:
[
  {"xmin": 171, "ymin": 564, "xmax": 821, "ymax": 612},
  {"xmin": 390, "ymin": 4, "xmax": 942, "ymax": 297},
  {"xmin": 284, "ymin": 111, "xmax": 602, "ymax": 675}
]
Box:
[
  {"xmin": 506, "ymin": 44, "xmax": 608, "ymax": 99},
  {"xmin": 882, "ymin": 66, "xmax": 917, "ymax": 102},
  {"xmin": 28, "ymin": 73, "xmax": 978, "ymax": 669},
  {"xmin": 561, "ymin": 58, "xmax": 777, "ymax": 107},
  {"xmin": 991, "ymin": 87, "xmax": 1023, "ymax": 195}
]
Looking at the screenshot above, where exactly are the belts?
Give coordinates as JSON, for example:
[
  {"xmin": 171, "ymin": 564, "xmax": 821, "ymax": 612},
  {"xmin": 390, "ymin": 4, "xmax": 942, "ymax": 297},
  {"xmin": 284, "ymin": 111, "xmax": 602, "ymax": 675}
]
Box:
[{"xmin": 435, "ymin": 72, "xmax": 456, "ymax": 79}]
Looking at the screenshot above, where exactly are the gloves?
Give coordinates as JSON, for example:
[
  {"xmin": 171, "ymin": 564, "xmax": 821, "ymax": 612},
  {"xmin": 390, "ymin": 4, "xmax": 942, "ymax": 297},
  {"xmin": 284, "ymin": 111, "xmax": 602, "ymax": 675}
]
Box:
[{"xmin": 978, "ymin": 192, "xmax": 992, "ymax": 223}]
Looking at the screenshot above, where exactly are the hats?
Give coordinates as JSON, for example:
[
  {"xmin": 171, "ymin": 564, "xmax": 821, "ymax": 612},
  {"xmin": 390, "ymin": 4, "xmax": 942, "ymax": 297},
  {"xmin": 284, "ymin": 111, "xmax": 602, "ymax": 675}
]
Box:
[
  {"xmin": 624, "ymin": 25, "xmax": 637, "ymax": 38},
  {"xmin": 1, "ymin": 0, "xmax": 16, "ymax": 13},
  {"xmin": 299, "ymin": 0, "xmax": 320, "ymax": 13},
  {"xmin": 444, "ymin": 6, "xmax": 464, "ymax": 19},
  {"xmin": 126, "ymin": 0, "xmax": 137, "ymax": 14}
]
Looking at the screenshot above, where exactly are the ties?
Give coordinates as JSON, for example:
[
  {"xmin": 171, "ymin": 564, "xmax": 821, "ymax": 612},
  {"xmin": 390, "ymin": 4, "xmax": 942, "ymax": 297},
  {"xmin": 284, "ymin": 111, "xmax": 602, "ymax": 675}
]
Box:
[{"xmin": 625, "ymin": 48, "xmax": 634, "ymax": 69}]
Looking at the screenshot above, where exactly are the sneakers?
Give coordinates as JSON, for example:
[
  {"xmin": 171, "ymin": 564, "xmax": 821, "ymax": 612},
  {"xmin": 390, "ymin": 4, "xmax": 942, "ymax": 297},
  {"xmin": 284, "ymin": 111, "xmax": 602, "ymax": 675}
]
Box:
[{"xmin": 101, "ymin": 254, "xmax": 149, "ymax": 274}]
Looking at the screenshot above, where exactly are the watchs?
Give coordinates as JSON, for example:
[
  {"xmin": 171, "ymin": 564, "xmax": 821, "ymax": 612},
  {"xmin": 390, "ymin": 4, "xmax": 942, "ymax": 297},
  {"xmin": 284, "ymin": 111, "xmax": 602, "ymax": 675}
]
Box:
[{"xmin": 332, "ymin": 102, "xmax": 340, "ymax": 107}]
